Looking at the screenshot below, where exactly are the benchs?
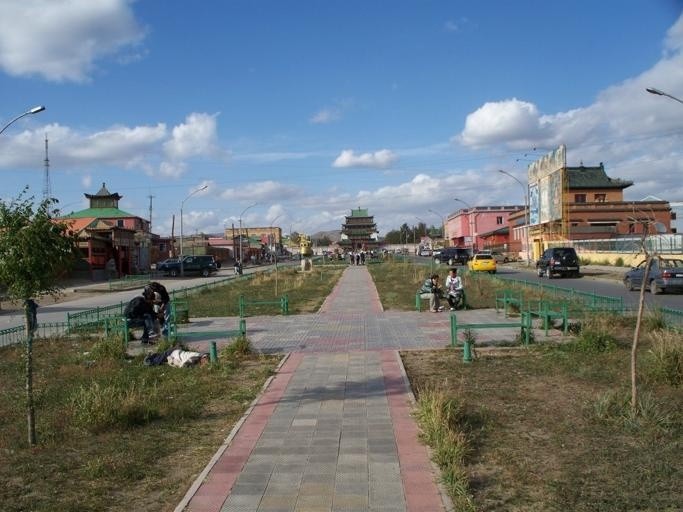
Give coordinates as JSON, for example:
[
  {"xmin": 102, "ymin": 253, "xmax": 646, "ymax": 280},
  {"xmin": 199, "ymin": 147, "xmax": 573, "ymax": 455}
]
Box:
[
  {"xmin": 168, "ymin": 302, "xmax": 189, "ymax": 322},
  {"xmin": 451, "ymin": 313, "xmax": 531, "ymax": 346},
  {"xmin": 415, "ymin": 290, "xmax": 467, "ymax": 312},
  {"xmin": 526, "ymin": 300, "xmax": 568, "ymax": 336},
  {"xmin": 104, "ymin": 317, "xmax": 177, "ymax": 347},
  {"xmin": 240, "ymin": 294, "xmax": 288, "ymax": 316},
  {"xmin": 495, "ymin": 289, "xmax": 523, "ymax": 315}
]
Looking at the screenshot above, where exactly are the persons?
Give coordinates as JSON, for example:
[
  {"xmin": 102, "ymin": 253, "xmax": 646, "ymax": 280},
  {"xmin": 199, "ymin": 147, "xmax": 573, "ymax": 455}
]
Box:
[
  {"xmin": 123, "ymin": 282, "xmax": 210, "ymax": 369},
  {"xmin": 340, "ymin": 247, "xmax": 374, "ymax": 266},
  {"xmin": 420, "ymin": 274, "xmax": 443, "ymax": 313},
  {"xmin": 232, "ymin": 258, "xmax": 239, "ymax": 275},
  {"xmin": 216, "ymin": 256, "xmax": 222, "ymax": 268},
  {"xmin": 444, "ymin": 268, "xmax": 463, "ymax": 311}
]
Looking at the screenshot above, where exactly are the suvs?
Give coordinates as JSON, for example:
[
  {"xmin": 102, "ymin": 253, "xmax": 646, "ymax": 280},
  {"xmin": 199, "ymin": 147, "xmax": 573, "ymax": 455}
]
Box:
[
  {"xmin": 534, "ymin": 246, "xmax": 579, "ymax": 280},
  {"xmin": 162, "ymin": 255, "xmax": 218, "ymax": 278},
  {"xmin": 431, "ymin": 245, "xmax": 470, "ymax": 266}
]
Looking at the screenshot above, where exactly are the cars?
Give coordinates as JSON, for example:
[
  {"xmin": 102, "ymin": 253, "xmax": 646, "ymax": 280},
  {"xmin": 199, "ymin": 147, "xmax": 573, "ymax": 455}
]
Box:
[
  {"xmin": 621, "ymin": 257, "xmax": 682, "ymax": 296},
  {"xmin": 466, "ymin": 253, "xmax": 498, "ymax": 275},
  {"xmin": 157, "ymin": 257, "xmax": 179, "ymax": 271},
  {"xmin": 476, "ymin": 249, "xmax": 509, "ymax": 264},
  {"xmin": 394, "ymin": 245, "xmax": 442, "ymax": 258}
]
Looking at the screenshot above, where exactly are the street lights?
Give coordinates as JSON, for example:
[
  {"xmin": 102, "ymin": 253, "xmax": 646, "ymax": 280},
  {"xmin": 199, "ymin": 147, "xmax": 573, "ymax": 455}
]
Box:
[
  {"xmin": 497, "ymin": 168, "xmax": 530, "ymax": 268},
  {"xmin": 178, "ymin": 184, "xmax": 209, "ymax": 277},
  {"xmin": 238, "ymin": 200, "xmax": 293, "ymax": 269},
  {"xmin": 0, "ymin": 103, "xmax": 46, "ymax": 135},
  {"xmin": 412, "ymin": 197, "xmax": 477, "ymax": 250},
  {"xmin": 643, "ymin": 84, "xmax": 683, "ymax": 104}
]
